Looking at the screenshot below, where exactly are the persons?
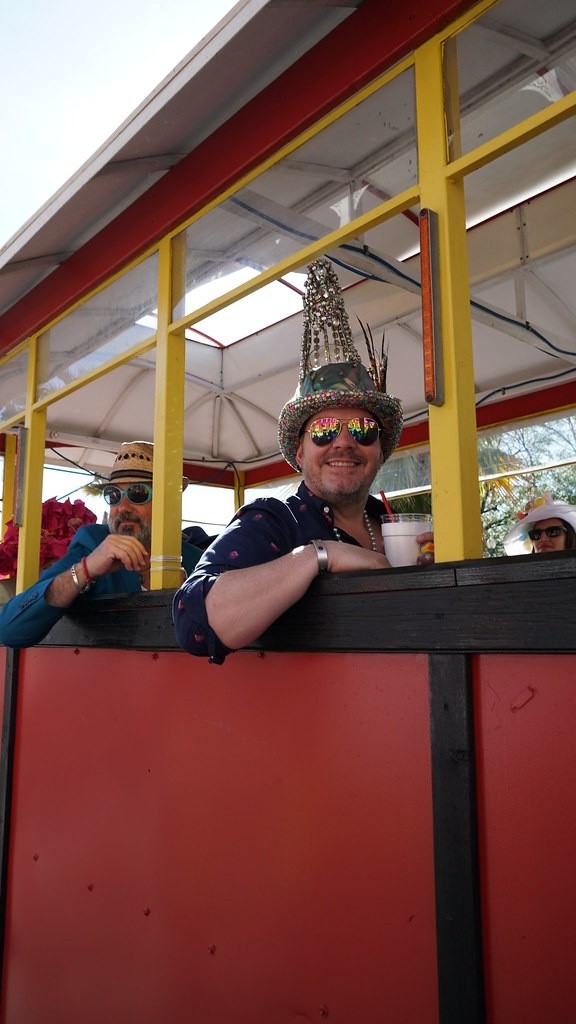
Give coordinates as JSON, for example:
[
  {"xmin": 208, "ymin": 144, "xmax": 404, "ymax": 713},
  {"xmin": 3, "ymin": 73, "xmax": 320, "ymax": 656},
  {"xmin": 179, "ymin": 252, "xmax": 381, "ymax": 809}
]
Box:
[
  {"xmin": 504, "ymin": 492, "xmax": 576, "ymax": 556},
  {"xmin": 171, "ymin": 362, "xmax": 435, "ymax": 664},
  {"xmin": 0, "ymin": 441, "xmax": 217, "ymax": 649}
]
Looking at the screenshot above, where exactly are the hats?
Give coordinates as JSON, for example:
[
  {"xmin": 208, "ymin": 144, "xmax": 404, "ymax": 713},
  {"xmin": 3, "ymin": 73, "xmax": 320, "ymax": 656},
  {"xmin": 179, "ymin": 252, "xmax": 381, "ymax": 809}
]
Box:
[
  {"xmin": 91, "ymin": 441, "xmax": 188, "ymax": 493},
  {"xmin": 503, "ymin": 492, "xmax": 576, "ymax": 555},
  {"xmin": 278, "ymin": 258, "xmax": 403, "ymax": 473}
]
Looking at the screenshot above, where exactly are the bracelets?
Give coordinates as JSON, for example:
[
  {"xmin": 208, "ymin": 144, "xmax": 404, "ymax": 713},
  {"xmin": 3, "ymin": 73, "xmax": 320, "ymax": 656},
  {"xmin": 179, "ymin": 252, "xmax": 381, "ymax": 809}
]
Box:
[
  {"xmin": 82, "ymin": 557, "xmax": 90, "ymax": 581},
  {"xmin": 71, "ymin": 565, "xmax": 83, "ymax": 592},
  {"xmin": 309, "ymin": 539, "xmax": 328, "ymax": 574}
]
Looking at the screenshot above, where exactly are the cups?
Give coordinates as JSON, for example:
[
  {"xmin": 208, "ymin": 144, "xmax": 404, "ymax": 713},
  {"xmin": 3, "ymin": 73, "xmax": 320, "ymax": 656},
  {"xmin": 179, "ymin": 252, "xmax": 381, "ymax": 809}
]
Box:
[{"xmin": 381, "ymin": 514, "xmax": 432, "ymax": 567}]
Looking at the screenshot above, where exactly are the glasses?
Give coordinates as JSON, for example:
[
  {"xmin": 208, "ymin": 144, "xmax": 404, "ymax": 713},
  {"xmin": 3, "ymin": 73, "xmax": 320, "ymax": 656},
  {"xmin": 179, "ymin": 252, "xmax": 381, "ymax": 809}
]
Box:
[
  {"xmin": 305, "ymin": 417, "xmax": 382, "ymax": 446},
  {"xmin": 102, "ymin": 484, "xmax": 152, "ymax": 506},
  {"xmin": 528, "ymin": 526, "xmax": 567, "ymax": 540}
]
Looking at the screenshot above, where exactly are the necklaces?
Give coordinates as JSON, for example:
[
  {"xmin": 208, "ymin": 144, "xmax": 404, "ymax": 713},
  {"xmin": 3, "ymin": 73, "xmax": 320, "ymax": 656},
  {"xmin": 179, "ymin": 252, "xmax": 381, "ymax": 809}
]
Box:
[{"xmin": 334, "ymin": 510, "xmax": 377, "ymax": 552}]
